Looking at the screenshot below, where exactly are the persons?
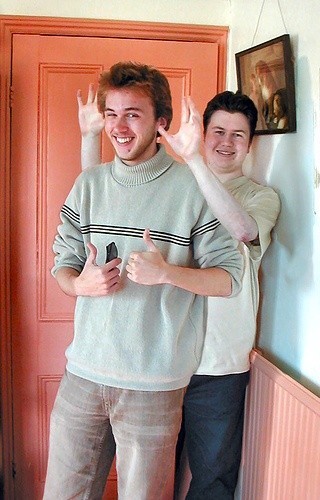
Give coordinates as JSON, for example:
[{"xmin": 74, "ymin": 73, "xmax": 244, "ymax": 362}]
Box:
[
  {"xmin": 42, "ymin": 61, "xmax": 246, "ymax": 500},
  {"xmin": 248, "ymin": 59, "xmax": 290, "ymax": 129},
  {"xmin": 76, "ymin": 81, "xmax": 281, "ymax": 500}
]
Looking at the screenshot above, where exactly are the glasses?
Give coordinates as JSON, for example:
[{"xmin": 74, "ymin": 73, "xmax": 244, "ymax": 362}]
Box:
[{"xmin": 105, "ymin": 242, "xmax": 118, "ymax": 272}]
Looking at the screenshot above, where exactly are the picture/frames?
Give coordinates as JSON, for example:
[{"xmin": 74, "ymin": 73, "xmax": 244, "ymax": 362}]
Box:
[{"xmin": 235, "ymin": 34, "xmax": 297, "ymax": 137}]
